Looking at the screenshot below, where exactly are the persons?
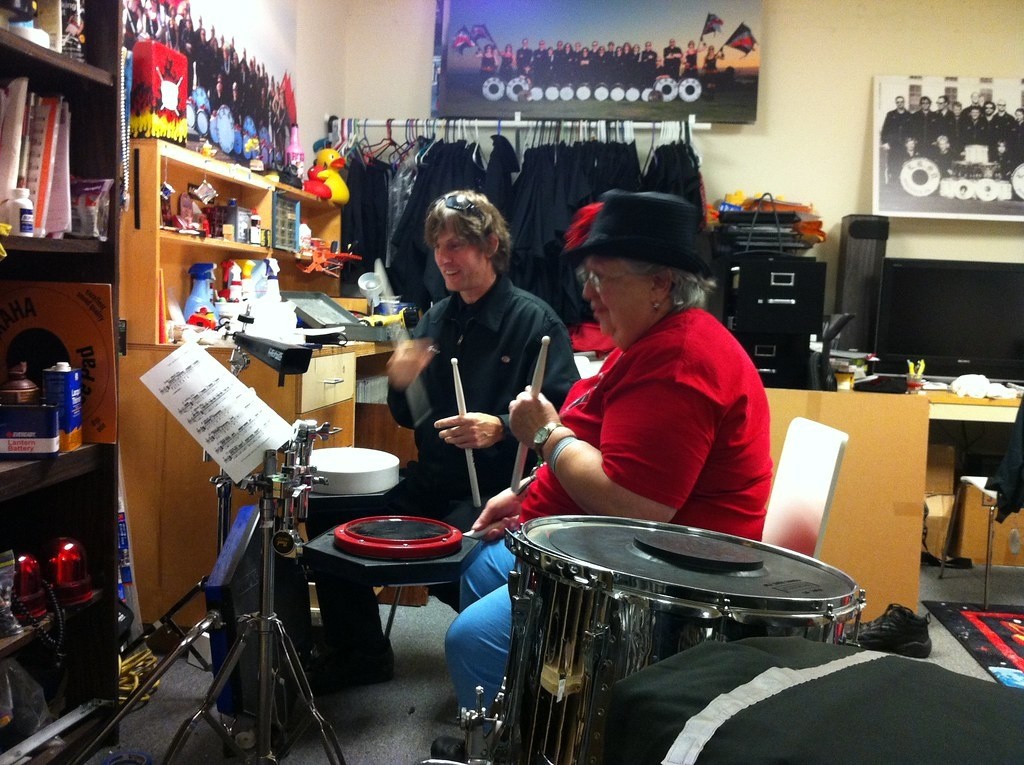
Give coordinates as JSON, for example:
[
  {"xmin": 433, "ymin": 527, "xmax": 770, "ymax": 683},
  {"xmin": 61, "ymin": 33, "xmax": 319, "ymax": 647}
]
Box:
[
  {"xmin": 122, "ymin": 0, "xmax": 287, "ymax": 171},
  {"xmin": 299, "ymin": 190, "xmax": 582, "ymax": 695},
  {"xmin": 880, "ymin": 91, "xmax": 1024, "ymax": 204},
  {"xmin": 474, "ymin": 39, "xmax": 725, "ymax": 96},
  {"xmin": 430, "ymin": 189, "xmax": 772, "ymax": 765}
]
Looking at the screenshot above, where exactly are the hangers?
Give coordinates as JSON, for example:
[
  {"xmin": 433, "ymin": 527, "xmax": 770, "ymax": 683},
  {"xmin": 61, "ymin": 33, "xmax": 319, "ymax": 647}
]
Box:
[{"xmin": 304, "ymin": 113, "xmax": 704, "ymax": 179}]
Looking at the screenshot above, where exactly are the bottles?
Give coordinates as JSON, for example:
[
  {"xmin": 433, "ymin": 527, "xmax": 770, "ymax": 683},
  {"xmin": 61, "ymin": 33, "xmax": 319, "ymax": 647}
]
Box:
[
  {"xmin": 7, "ymin": 188, "xmax": 34, "ymax": 237},
  {"xmin": 0, "ymin": 362, "xmax": 42, "ymax": 405}
]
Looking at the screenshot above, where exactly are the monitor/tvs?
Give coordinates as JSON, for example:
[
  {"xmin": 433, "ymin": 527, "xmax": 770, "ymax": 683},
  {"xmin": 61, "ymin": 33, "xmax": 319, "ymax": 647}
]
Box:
[{"xmin": 875, "ymin": 254, "xmax": 1024, "ymax": 382}]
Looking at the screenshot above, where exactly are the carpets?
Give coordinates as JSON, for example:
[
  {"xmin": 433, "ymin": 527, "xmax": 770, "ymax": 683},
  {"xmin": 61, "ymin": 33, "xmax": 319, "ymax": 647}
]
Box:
[{"xmin": 920, "ymin": 600, "xmax": 1024, "ymax": 689}]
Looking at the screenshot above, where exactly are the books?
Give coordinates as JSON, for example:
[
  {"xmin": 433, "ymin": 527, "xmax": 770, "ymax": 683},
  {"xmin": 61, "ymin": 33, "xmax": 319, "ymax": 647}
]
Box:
[{"xmin": 0, "ymin": 77, "xmax": 71, "ymax": 237}]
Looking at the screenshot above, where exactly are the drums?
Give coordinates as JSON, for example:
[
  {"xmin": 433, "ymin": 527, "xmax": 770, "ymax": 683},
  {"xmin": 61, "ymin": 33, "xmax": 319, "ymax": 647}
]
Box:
[
  {"xmin": 333, "ymin": 512, "xmax": 463, "ymax": 560},
  {"xmin": 294, "ymin": 446, "xmax": 400, "ymax": 494},
  {"xmin": 500, "ymin": 514, "xmax": 861, "ymax": 765}
]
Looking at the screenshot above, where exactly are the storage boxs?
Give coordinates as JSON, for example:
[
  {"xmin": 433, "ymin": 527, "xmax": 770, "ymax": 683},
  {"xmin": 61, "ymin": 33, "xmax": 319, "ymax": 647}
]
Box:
[{"xmin": 920, "ymin": 433, "xmax": 1024, "ymax": 567}]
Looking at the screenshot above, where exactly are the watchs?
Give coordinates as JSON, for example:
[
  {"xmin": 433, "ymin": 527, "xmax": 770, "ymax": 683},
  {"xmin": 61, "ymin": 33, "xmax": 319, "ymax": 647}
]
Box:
[{"xmin": 533, "ymin": 422, "xmax": 564, "ymax": 460}]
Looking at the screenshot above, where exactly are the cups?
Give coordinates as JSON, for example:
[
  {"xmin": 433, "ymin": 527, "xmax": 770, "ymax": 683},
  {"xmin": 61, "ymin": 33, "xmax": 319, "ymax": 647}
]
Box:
[{"xmin": 907, "ymin": 373, "xmax": 922, "ymax": 391}]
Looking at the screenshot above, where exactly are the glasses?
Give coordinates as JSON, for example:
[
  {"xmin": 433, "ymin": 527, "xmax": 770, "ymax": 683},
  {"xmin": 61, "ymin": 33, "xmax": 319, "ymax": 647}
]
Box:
[
  {"xmin": 998, "ymin": 104, "xmax": 1006, "ymax": 106},
  {"xmin": 897, "ymin": 101, "xmax": 903, "ymax": 103},
  {"xmin": 922, "ymin": 102, "xmax": 930, "ymax": 105},
  {"xmin": 575, "ymin": 266, "xmax": 659, "ymax": 294},
  {"xmin": 937, "ymin": 101, "xmax": 945, "ymax": 105},
  {"xmin": 985, "ymin": 107, "xmax": 993, "ymax": 109},
  {"xmin": 433, "ymin": 195, "xmax": 486, "ymax": 222}
]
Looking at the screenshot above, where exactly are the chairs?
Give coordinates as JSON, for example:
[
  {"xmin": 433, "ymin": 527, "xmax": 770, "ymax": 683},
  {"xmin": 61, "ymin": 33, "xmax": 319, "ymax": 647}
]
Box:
[
  {"xmin": 938, "ymin": 391, "xmax": 1024, "ymax": 612},
  {"xmin": 761, "ymin": 415, "xmax": 851, "ymax": 561}
]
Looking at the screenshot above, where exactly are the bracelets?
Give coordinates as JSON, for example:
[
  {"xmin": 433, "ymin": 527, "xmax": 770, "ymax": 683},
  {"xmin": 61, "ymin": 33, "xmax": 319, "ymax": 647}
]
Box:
[{"xmin": 549, "ymin": 435, "xmax": 578, "ymax": 473}]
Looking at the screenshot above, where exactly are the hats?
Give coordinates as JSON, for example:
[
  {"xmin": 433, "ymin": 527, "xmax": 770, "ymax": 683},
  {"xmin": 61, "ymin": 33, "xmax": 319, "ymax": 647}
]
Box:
[
  {"xmin": 571, "ymin": 189, "xmax": 712, "ymax": 280},
  {"xmin": 232, "ymin": 82, "xmax": 238, "ymax": 90}
]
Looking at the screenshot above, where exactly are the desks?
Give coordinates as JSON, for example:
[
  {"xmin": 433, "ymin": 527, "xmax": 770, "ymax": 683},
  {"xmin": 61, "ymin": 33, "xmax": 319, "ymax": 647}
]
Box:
[
  {"xmin": 763, "ymin": 396, "xmax": 929, "ymax": 628},
  {"xmin": 835, "ymin": 371, "xmax": 1024, "ymax": 423}
]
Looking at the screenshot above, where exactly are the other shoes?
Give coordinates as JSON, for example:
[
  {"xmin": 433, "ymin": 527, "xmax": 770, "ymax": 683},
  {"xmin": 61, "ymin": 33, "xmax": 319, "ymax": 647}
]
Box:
[
  {"xmin": 305, "ymin": 637, "xmax": 394, "ymax": 694},
  {"xmin": 431, "ymin": 736, "xmax": 464, "ymax": 762}
]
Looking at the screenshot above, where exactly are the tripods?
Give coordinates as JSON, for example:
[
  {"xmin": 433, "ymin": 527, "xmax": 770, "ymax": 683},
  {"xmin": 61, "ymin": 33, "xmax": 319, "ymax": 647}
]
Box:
[{"xmin": 161, "ymin": 495, "xmax": 350, "ymax": 765}]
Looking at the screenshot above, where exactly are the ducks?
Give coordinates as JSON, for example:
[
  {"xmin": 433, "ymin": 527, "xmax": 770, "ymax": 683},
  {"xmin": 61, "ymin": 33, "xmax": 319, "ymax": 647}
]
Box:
[{"xmin": 302, "ymin": 148, "xmax": 350, "ymax": 205}]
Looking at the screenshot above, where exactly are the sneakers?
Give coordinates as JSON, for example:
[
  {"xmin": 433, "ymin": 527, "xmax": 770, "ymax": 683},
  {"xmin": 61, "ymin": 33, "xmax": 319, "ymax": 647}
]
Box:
[{"xmin": 839, "ymin": 603, "xmax": 932, "ymax": 658}]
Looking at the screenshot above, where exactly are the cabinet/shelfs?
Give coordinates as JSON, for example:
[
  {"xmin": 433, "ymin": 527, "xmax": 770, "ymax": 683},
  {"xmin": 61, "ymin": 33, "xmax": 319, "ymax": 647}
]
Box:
[
  {"xmin": 0, "ymin": 0, "xmax": 124, "ymax": 765},
  {"xmin": 116, "ymin": 138, "xmax": 357, "ymax": 644}
]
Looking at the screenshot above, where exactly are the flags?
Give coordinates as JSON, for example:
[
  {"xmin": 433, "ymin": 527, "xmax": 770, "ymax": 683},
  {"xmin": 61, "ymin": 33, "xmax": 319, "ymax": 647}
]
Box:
[
  {"xmin": 281, "ymin": 74, "xmax": 296, "ymax": 126},
  {"xmin": 471, "ymin": 24, "xmax": 493, "ymax": 41},
  {"xmin": 725, "ymin": 23, "xmax": 756, "ymax": 54},
  {"xmin": 701, "ymin": 14, "xmax": 725, "ymax": 36},
  {"xmin": 451, "ymin": 26, "xmax": 476, "ymax": 54}
]
